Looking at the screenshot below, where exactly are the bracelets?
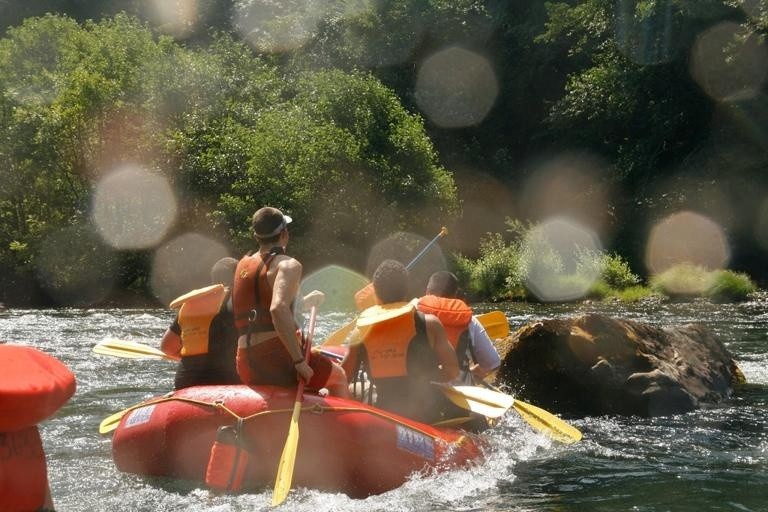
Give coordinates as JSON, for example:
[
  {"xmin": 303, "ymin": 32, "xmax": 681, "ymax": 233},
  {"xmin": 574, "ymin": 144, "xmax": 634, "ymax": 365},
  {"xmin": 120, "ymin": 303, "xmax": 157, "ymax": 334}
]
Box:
[{"xmin": 292, "ymin": 357, "xmax": 304, "ymax": 364}]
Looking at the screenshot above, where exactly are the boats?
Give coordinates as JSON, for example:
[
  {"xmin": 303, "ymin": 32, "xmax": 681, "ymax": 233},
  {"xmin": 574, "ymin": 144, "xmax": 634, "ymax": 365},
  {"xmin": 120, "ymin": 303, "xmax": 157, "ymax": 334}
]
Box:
[{"xmin": 112, "ymin": 384, "xmax": 485, "ymax": 500}]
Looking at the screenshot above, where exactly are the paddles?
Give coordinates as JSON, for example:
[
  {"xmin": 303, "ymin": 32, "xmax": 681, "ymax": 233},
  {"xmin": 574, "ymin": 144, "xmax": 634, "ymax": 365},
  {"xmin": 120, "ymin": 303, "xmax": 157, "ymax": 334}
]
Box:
[
  {"xmin": 92, "ymin": 337, "xmax": 182, "ymax": 362},
  {"xmin": 100, "ymin": 406, "xmax": 141, "ymax": 433},
  {"xmin": 272, "ymin": 301, "xmax": 316, "ymax": 505},
  {"xmin": 475, "ymin": 379, "xmax": 581, "ymax": 446},
  {"xmin": 320, "ymin": 228, "xmax": 448, "ymax": 347},
  {"xmin": 476, "ymin": 312, "xmax": 509, "ymax": 339},
  {"xmin": 310, "ymin": 348, "xmax": 513, "ymax": 418}
]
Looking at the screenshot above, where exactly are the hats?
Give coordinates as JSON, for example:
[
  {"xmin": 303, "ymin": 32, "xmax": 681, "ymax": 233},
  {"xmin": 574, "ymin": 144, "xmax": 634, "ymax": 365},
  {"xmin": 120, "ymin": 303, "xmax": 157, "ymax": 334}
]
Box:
[{"xmin": 251, "ymin": 206, "xmax": 293, "ymax": 238}]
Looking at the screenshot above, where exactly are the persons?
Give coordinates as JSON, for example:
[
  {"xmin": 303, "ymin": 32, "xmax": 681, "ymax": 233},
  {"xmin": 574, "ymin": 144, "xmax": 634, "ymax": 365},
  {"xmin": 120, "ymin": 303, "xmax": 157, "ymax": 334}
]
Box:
[
  {"xmin": 410, "ymin": 270, "xmax": 501, "ymax": 384},
  {"xmin": 338, "ymin": 259, "xmax": 461, "ymax": 426},
  {"xmin": 159, "ymin": 257, "xmax": 242, "ymax": 386},
  {"xmin": 232, "ymin": 207, "xmax": 351, "ymax": 401}
]
